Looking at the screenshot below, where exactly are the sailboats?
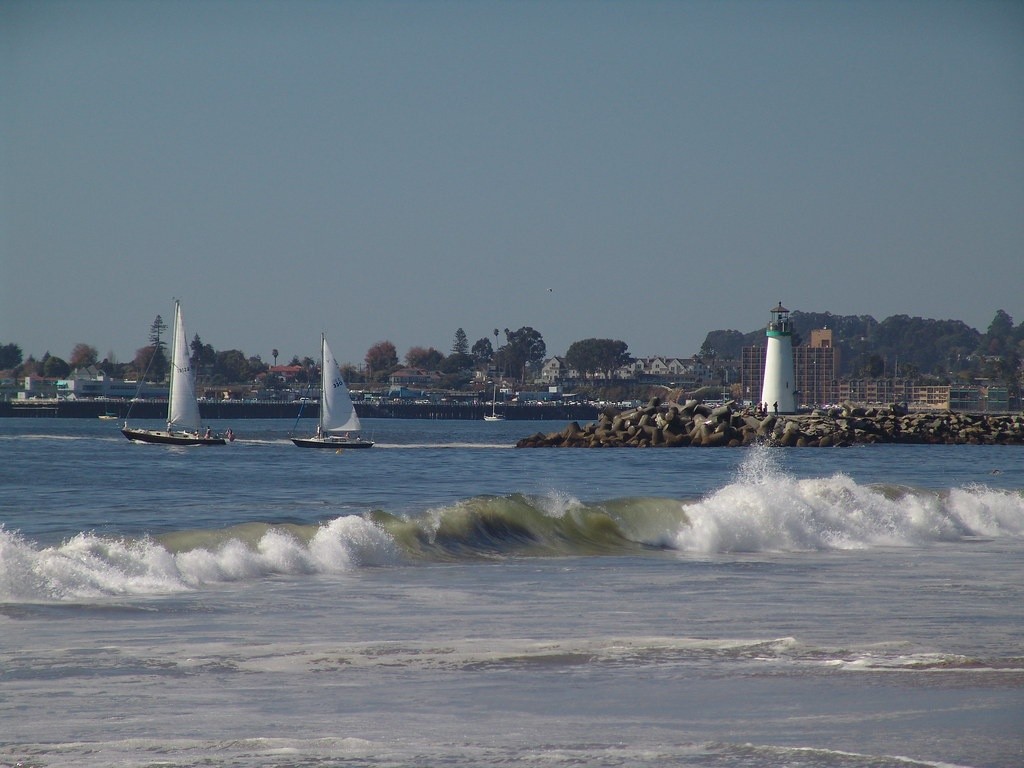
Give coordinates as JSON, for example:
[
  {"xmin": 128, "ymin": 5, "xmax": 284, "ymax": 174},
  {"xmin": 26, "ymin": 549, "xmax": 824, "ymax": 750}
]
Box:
[
  {"xmin": 290, "ymin": 332, "xmax": 375, "ymax": 449},
  {"xmin": 484, "ymin": 383, "xmax": 506, "ymax": 422},
  {"xmin": 121, "ymin": 300, "xmax": 226, "ymax": 445}
]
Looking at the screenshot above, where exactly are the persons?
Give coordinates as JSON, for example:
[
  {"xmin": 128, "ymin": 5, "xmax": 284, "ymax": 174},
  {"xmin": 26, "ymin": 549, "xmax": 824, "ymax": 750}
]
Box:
[
  {"xmin": 316, "ymin": 424, "xmax": 321, "ymax": 439},
  {"xmin": 346, "ymin": 431, "xmax": 351, "ymax": 437},
  {"xmin": 357, "ymin": 436, "xmax": 361, "ymax": 441},
  {"xmin": 773, "ymin": 401, "xmax": 779, "ymax": 416},
  {"xmin": 166, "ymin": 421, "xmax": 172, "ymax": 434},
  {"xmin": 757, "ymin": 401, "xmax": 768, "ymax": 413},
  {"xmin": 194, "ymin": 429, "xmax": 200, "ymax": 438},
  {"xmin": 206, "ymin": 426, "xmax": 211, "ymax": 438}
]
